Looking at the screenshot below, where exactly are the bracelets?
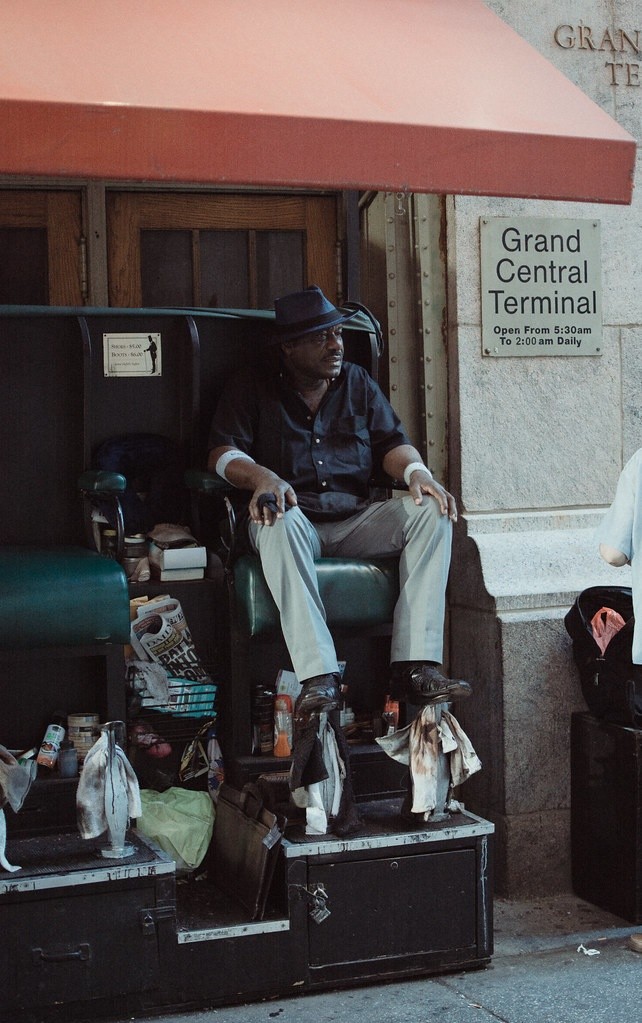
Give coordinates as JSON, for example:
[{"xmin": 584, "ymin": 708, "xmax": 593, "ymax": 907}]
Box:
[
  {"xmin": 402, "ymin": 461, "xmax": 433, "ymax": 485},
  {"xmin": 216, "ymin": 450, "xmax": 256, "ymax": 489}
]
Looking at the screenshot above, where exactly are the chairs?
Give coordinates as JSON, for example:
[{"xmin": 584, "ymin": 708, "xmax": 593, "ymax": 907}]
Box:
[
  {"xmin": 177, "ymin": 317, "xmax": 451, "ymax": 756},
  {"xmin": 0, "ymin": 314, "xmax": 134, "ymax": 746}
]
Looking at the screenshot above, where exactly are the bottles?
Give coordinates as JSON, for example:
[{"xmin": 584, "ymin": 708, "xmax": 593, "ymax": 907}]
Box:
[
  {"xmin": 100, "ymin": 529, "xmax": 145, "ymax": 575},
  {"xmin": 248, "ymin": 684, "xmax": 398, "ymax": 758},
  {"xmin": 35, "ymin": 709, "xmax": 100, "ymax": 778}
]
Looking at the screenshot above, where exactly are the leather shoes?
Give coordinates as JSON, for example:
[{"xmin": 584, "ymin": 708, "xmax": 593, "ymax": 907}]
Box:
[
  {"xmin": 296, "ymin": 673, "xmax": 342, "ymax": 711},
  {"xmin": 389, "ymin": 661, "xmax": 473, "ymax": 706}
]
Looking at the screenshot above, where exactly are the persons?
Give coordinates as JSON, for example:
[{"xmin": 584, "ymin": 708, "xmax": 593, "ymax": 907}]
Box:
[
  {"xmin": 205, "ymin": 284, "xmax": 472, "ymax": 713},
  {"xmin": 597, "ymin": 448, "xmax": 642, "ymax": 957}
]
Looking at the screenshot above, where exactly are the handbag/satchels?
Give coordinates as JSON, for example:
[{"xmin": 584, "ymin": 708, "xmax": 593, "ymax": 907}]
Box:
[{"xmin": 591, "ymin": 606, "xmax": 626, "ymax": 656}]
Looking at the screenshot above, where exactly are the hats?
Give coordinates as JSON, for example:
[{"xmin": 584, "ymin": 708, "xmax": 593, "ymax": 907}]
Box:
[{"xmin": 257, "ymin": 284, "xmax": 360, "ymax": 345}]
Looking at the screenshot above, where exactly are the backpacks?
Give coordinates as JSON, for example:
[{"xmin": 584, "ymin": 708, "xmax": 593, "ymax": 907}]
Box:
[{"xmin": 564, "ymin": 586, "xmax": 642, "ymax": 730}]
